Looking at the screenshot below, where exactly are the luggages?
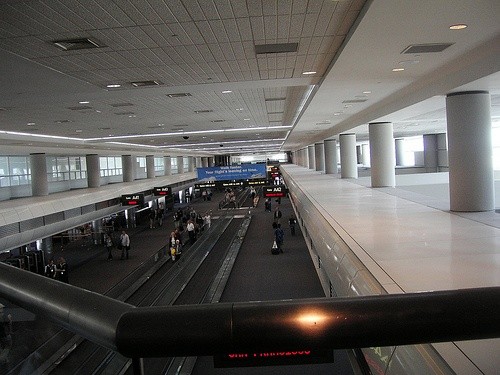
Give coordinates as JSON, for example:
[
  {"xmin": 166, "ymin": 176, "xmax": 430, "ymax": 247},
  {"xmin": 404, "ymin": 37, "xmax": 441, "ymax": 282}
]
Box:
[{"xmin": 271, "ymin": 240, "xmax": 280, "ymax": 255}]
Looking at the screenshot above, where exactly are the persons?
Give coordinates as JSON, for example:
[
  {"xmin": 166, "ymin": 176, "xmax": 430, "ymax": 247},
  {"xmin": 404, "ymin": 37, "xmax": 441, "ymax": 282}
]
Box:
[
  {"xmin": 274, "ymin": 206, "xmax": 282, "ymax": 224},
  {"xmin": 104, "ymin": 235, "xmax": 115, "ymax": 263},
  {"xmin": 202, "ymin": 189, "xmax": 212, "ymax": 201},
  {"xmin": 0, "ymin": 302, "xmax": 13, "ymax": 349},
  {"xmin": 169, "ymin": 228, "xmax": 183, "ymax": 262},
  {"xmin": 205, "ymin": 212, "xmax": 211, "ymax": 229},
  {"xmin": 148, "ymin": 207, "xmax": 155, "ymax": 229},
  {"xmin": 288, "ymin": 215, "xmax": 296, "ymax": 237},
  {"xmin": 182, "ymin": 205, "xmax": 203, "ymax": 246},
  {"xmin": 265, "ymin": 199, "xmax": 271, "ymax": 212},
  {"xmin": 119, "ymin": 230, "xmax": 130, "ymax": 260},
  {"xmin": 224, "ymin": 188, "xmax": 234, "ymax": 202},
  {"xmin": 173, "ymin": 209, "xmax": 183, "ymax": 229},
  {"xmin": 156, "ymin": 208, "xmax": 164, "ymax": 227},
  {"xmin": 56, "ymin": 254, "xmax": 69, "ymax": 283},
  {"xmin": 44, "ymin": 258, "xmax": 56, "ymax": 279},
  {"xmin": 252, "ymin": 192, "xmax": 256, "ymax": 199},
  {"xmin": 274, "ymin": 223, "xmax": 284, "ymax": 253}
]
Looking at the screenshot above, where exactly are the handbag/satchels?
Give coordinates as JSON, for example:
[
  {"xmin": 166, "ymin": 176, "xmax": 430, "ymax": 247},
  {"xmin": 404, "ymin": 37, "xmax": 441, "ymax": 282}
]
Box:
[{"xmin": 116, "ymin": 242, "xmax": 123, "ymax": 250}]
